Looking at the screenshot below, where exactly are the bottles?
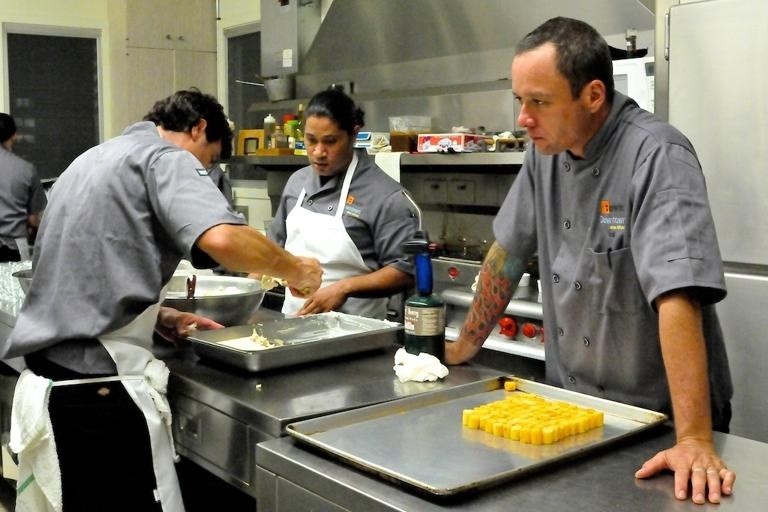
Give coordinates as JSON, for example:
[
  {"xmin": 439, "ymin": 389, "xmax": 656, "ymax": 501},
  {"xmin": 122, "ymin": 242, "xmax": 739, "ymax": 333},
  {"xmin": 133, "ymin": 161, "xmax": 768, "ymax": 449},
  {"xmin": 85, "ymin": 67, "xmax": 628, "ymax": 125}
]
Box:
[
  {"xmin": 403, "ymin": 252, "xmax": 446, "ymax": 367},
  {"xmin": 625, "ymin": 28, "xmax": 636, "ymax": 51},
  {"xmin": 264, "ymin": 103, "xmax": 307, "ymax": 150}
]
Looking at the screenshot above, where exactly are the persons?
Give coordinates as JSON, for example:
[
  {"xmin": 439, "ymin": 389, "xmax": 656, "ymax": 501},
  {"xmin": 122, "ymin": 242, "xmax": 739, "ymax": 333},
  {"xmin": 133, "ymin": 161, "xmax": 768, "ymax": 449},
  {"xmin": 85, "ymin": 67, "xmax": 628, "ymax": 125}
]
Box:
[
  {"xmin": 445, "ymin": 12, "xmax": 737, "ymax": 507},
  {"xmin": 0, "ymin": 112, "xmax": 49, "ymax": 262},
  {"xmin": 265, "ymin": 81, "xmax": 423, "ymax": 321},
  {"xmin": 1, "ymin": 84, "xmax": 324, "ymax": 512}
]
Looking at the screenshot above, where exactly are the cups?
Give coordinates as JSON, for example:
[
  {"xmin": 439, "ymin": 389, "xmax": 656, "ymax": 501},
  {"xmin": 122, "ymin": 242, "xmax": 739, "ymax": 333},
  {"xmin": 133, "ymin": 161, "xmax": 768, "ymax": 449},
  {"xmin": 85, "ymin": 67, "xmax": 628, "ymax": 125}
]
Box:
[{"xmin": 518, "ymin": 273, "xmax": 530, "ymax": 287}]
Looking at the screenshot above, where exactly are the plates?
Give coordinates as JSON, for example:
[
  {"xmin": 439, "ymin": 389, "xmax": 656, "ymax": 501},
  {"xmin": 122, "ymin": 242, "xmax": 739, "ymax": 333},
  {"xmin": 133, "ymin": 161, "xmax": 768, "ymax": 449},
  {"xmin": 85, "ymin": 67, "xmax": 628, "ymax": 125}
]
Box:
[{"xmin": 606, "ymin": 44, "xmax": 649, "ymax": 59}]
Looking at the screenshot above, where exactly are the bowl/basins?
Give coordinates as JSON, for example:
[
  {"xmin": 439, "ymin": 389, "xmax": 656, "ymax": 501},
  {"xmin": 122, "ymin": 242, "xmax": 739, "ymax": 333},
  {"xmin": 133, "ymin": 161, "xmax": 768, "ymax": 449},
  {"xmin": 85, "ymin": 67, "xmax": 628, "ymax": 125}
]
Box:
[
  {"xmin": 158, "ymin": 259, "xmax": 267, "ymax": 327},
  {"xmin": 10, "ymin": 269, "xmax": 36, "ymax": 295}
]
